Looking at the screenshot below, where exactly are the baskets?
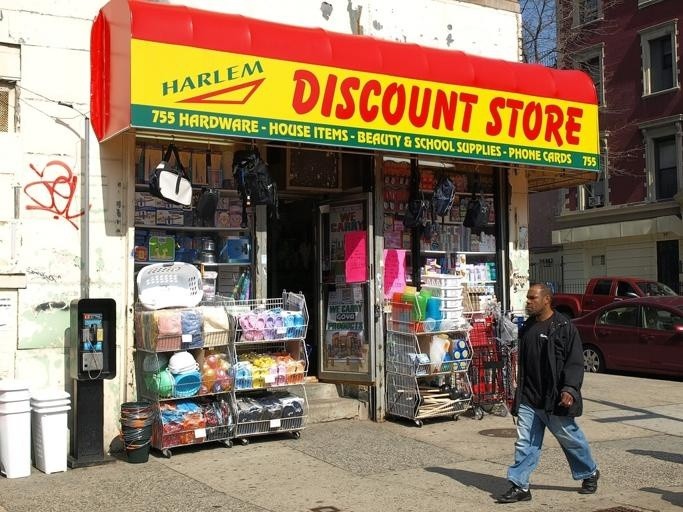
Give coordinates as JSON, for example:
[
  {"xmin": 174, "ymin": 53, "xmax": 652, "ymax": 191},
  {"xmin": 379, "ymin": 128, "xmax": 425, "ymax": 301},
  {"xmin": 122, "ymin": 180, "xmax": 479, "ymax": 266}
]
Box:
[
  {"xmin": 420, "ymin": 273, "xmax": 464, "ymax": 320},
  {"xmin": 137, "ymin": 262, "xmax": 204, "ymax": 311}
]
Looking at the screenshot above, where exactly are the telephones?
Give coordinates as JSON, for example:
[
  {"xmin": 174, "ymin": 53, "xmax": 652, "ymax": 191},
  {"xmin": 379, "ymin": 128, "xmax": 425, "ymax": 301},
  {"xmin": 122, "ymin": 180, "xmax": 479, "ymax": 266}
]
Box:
[{"xmin": 81, "ymin": 313, "xmax": 104, "ymax": 371}]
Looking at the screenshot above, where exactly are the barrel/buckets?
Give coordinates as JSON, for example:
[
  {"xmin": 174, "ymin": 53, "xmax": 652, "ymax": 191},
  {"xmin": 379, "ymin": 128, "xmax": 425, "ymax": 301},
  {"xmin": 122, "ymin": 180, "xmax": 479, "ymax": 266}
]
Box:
[
  {"xmin": 120, "ymin": 402, "xmax": 154, "ymax": 463},
  {"xmin": 429, "ymin": 334, "xmax": 451, "ymax": 372}
]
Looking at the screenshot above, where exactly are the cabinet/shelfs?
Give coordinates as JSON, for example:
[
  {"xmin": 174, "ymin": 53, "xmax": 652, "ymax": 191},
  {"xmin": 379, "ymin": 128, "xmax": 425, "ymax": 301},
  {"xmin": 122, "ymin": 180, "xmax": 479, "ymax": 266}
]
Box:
[
  {"xmin": 137, "ymin": 289, "xmax": 236, "ymax": 456},
  {"xmin": 235, "ymin": 292, "xmax": 310, "ymax": 444},
  {"xmin": 385, "ymin": 301, "xmax": 473, "ymax": 427},
  {"xmin": 135, "ymin": 182, "xmax": 256, "ymax": 301}
]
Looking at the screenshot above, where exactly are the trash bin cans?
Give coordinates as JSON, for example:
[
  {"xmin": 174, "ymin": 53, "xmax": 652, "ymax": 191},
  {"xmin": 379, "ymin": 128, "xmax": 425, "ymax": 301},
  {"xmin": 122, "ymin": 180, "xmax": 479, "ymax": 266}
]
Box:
[{"xmin": 0, "ymin": 383, "xmax": 71, "ymax": 479}]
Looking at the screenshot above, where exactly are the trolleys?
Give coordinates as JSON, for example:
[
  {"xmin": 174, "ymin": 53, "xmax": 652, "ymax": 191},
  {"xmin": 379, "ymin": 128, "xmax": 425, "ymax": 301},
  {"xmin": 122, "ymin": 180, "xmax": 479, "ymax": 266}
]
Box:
[{"xmin": 473, "ymin": 343, "xmax": 510, "ymax": 421}]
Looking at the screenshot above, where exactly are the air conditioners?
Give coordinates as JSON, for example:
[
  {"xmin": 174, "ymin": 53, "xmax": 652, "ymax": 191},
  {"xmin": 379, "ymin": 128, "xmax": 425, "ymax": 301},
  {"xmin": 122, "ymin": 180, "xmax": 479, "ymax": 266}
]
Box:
[{"xmin": 589, "ymin": 196, "xmax": 601, "ymax": 206}]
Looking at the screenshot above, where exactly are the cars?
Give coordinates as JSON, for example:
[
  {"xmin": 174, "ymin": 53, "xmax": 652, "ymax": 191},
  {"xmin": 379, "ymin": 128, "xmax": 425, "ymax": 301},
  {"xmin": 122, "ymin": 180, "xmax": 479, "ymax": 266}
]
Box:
[{"xmin": 565, "ymin": 295, "xmax": 682, "ymax": 374}]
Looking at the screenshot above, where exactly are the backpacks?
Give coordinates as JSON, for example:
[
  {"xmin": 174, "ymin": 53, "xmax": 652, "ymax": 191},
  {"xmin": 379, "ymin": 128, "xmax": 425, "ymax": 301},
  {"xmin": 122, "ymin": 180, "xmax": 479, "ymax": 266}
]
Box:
[
  {"xmin": 232, "ymin": 150, "xmax": 274, "ymax": 206},
  {"xmin": 433, "ymin": 177, "xmax": 457, "ymax": 216}
]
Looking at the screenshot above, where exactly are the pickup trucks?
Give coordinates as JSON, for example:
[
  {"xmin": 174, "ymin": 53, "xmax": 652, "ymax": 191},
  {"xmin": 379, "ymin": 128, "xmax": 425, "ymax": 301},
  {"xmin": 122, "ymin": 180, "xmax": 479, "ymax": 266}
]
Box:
[{"xmin": 551, "ymin": 276, "xmax": 678, "ymax": 318}]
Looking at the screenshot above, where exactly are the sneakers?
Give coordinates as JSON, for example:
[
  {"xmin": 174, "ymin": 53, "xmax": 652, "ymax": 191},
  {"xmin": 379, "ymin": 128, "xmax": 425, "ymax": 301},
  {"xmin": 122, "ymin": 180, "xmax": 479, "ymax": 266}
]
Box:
[
  {"xmin": 579, "ymin": 470, "xmax": 599, "ymax": 493},
  {"xmin": 497, "ymin": 485, "xmax": 531, "ymax": 502}
]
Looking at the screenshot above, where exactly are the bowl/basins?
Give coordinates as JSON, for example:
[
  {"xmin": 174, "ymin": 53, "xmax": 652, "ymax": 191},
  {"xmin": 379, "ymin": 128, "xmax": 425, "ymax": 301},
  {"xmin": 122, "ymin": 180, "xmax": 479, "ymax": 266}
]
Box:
[{"xmin": 145, "ymin": 351, "xmax": 202, "ymax": 400}]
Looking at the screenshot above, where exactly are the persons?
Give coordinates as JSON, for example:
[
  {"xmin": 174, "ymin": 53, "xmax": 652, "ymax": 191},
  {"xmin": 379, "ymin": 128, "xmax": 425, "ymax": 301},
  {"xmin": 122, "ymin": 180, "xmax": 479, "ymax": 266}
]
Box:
[{"xmin": 496, "ymin": 283, "xmax": 600, "ymax": 503}]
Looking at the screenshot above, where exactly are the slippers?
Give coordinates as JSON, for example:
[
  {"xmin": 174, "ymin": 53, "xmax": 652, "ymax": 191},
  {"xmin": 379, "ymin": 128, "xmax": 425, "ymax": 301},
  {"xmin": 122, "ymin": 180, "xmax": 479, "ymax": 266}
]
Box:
[
  {"xmin": 235, "ymin": 351, "xmax": 304, "ymax": 388},
  {"xmin": 237, "ymin": 308, "xmax": 304, "ymax": 341}
]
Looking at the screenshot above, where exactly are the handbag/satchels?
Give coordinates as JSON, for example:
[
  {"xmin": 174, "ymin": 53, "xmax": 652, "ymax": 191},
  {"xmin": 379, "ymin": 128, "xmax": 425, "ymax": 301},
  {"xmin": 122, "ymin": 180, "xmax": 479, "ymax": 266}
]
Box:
[
  {"xmin": 149, "ymin": 163, "xmax": 193, "ymax": 205},
  {"xmin": 463, "ymin": 199, "xmax": 489, "ymax": 226},
  {"xmin": 404, "ymin": 207, "xmax": 428, "ymax": 228}
]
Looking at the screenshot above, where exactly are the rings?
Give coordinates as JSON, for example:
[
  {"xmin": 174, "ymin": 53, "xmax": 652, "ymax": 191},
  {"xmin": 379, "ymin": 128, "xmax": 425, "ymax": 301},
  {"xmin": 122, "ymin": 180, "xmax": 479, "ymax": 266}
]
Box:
[{"xmin": 568, "ymin": 402, "xmax": 570, "ymax": 405}]
